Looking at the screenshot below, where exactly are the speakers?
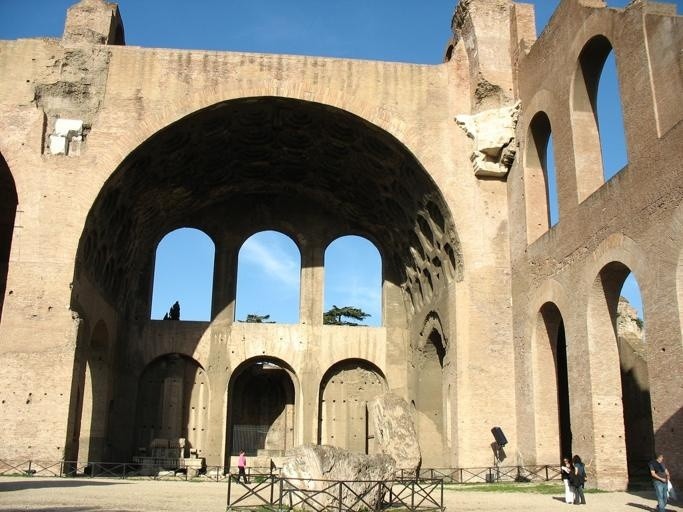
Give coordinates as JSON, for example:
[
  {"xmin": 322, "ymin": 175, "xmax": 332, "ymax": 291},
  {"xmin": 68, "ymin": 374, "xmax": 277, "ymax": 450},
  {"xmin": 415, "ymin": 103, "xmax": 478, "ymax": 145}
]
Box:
[{"xmin": 491, "ymin": 426, "xmax": 508, "ymax": 445}]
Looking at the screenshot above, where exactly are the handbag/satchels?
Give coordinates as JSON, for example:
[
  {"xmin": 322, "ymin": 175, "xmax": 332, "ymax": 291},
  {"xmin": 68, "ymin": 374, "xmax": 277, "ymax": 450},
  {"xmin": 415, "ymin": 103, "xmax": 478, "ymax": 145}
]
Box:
[{"xmin": 667, "ymin": 479, "xmax": 677, "ymax": 502}]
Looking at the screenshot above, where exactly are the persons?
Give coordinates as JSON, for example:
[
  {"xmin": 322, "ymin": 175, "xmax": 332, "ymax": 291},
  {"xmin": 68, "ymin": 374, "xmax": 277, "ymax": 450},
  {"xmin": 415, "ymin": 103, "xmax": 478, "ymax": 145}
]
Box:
[
  {"xmin": 568, "ymin": 454, "xmax": 586, "ymax": 503},
  {"xmin": 560, "ymin": 456, "xmax": 575, "ymax": 504},
  {"xmin": 234, "ymin": 448, "xmax": 251, "ymax": 483},
  {"xmin": 648, "ymin": 453, "xmax": 670, "ymax": 512}
]
